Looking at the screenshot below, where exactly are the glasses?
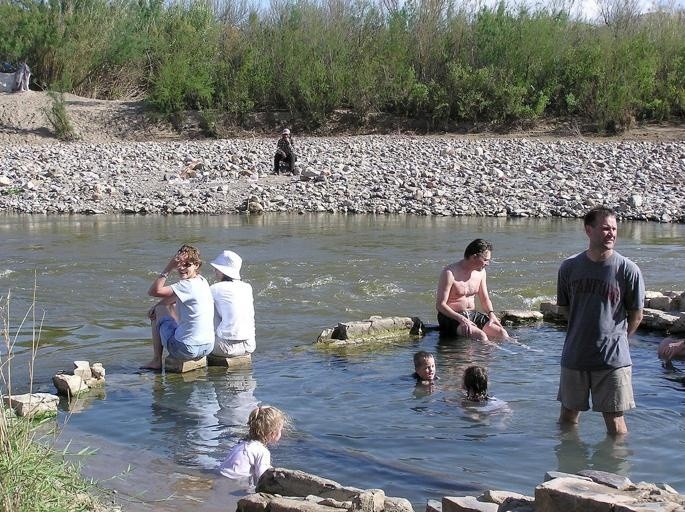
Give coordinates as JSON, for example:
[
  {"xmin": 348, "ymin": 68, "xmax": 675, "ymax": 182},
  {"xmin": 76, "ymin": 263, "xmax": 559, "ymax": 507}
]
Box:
[{"xmin": 183, "ymin": 263, "xmax": 193, "ymax": 268}]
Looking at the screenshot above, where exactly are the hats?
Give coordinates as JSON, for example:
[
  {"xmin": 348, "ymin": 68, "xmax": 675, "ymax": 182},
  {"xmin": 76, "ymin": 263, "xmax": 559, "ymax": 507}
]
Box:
[
  {"xmin": 282, "ymin": 128, "xmax": 291, "ymax": 135},
  {"xmin": 208, "ymin": 250, "xmax": 242, "ymax": 280}
]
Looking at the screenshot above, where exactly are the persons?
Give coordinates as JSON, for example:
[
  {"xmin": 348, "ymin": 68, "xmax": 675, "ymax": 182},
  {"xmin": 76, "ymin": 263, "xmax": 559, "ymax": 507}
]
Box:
[
  {"xmin": 208, "ymin": 249, "xmax": 256, "ymax": 358},
  {"xmin": 410, "ymin": 350, "xmax": 440, "ymax": 381},
  {"xmin": 436, "ymin": 238, "xmax": 544, "ymax": 356},
  {"xmin": 461, "ymin": 364, "xmax": 490, "ymax": 401},
  {"xmin": 556, "ymin": 205, "xmax": 646, "ymax": 430},
  {"xmin": 214, "ymin": 405, "xmax": 295, "ymax": 485},
  {"xmin": 270, "ymin": 128, "xmax": 297, "ymax": 176},
  {"xmin": 138, "ymin": 243, "xmax": 215, "ymax": 371}
]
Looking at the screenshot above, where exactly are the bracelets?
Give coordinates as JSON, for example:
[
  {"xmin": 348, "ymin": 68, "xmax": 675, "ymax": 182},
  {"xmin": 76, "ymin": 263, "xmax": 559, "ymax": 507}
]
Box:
[{"xmin": 159, "ymin": 272, "xmax": 168, "ymax": 279}]
